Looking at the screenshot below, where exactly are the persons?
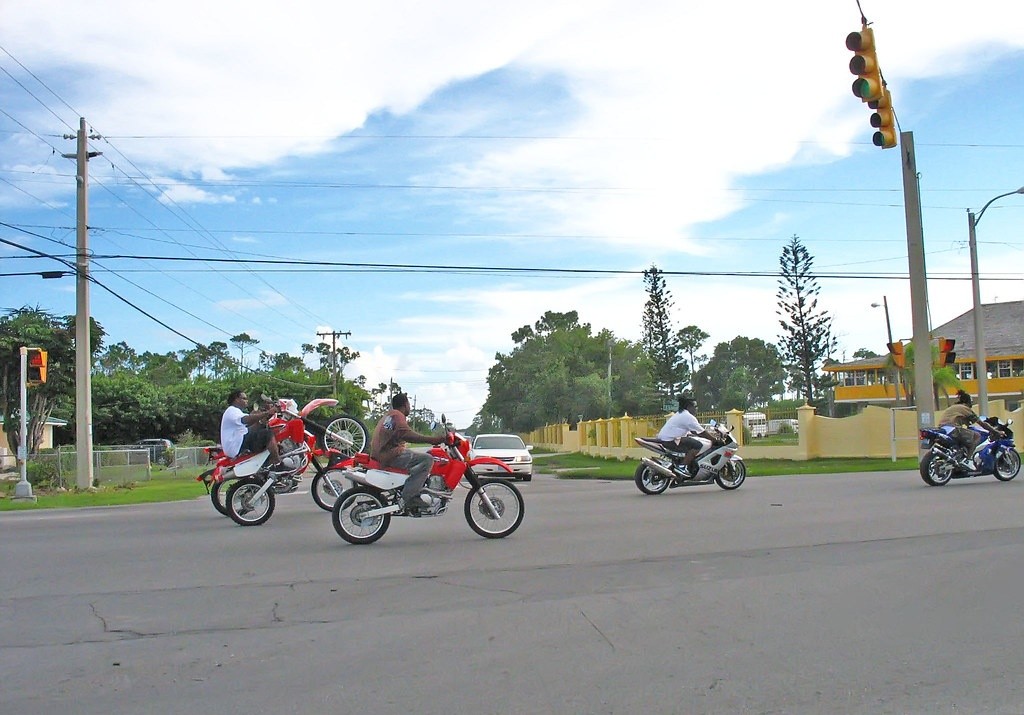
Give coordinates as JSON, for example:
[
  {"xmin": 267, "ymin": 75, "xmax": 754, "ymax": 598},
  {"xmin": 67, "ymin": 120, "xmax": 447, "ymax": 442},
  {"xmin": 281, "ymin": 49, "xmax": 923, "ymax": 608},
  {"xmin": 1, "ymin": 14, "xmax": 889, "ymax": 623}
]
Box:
[
  {"xmin": 220, "ymin": 389, "xmax": 294, "ymax": 471},
  {"xmin": 657, "ymin": 399, "xmax": 722, "ymax": 477},
  {"xmin": 938, "ymin": 390, "xmax": 1006, "ymax": 471},
  {"xmin": 372, "ymin": 393, "xmax": 451, "ymax": 508}
]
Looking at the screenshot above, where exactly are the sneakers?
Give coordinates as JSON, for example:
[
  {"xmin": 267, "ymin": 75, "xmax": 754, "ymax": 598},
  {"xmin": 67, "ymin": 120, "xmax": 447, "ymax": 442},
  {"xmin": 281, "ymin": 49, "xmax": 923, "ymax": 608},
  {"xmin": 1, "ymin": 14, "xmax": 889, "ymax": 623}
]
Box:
[
  {"xmin": 269, "ymin": 461, "xmax": 297, "ymax": 475},
  {"xmin": 962, "ymin": 457, "xmax": 977, "ymax": 470},
  {"xmin": 674, "ymin": 465, "xmax": 691, "ymax": 478},
  {"xmin": 405, "ymin": 496, "xmax": 430, "ymax": 507}
]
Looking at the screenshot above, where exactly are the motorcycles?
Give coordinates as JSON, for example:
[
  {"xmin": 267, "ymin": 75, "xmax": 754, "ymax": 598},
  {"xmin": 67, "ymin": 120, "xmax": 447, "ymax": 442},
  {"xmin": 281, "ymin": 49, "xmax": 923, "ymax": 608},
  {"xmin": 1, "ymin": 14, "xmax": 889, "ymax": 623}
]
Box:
[
  {"xmin": 331, "ymin": 413, "xmax": 526, "ymax": 546},
  {"xmin": 633, "ymin": 418, "xmax": 746, "ymax": 495},
  {"xmin": 195, "ymin": 393, "xmax": 369, "ymax": 527},
  {"xmin": 919, "ymin": 416, "xmax": 1021, "ymax": 486}
]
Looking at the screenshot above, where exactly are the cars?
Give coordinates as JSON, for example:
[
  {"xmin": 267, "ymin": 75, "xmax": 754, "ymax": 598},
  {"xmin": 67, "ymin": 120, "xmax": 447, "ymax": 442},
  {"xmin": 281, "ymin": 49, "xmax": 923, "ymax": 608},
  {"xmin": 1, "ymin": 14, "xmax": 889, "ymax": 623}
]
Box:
[
  {"xmin": 132, "ymin": 438, "xmax": 173, "ymax": 466},
  {"xmin": 468, "ymin": 433, "xmax": 534, "ymax": 481}
]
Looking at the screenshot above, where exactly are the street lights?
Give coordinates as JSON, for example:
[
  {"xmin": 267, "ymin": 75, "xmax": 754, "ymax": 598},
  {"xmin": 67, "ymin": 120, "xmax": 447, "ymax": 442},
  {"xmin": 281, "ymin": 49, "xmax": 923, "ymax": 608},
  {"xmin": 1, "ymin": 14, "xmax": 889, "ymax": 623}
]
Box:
[
  {"xmin": 967, "ymin": 187, "xmax": 1024, "ymax": 417},
  {"xmin": 870, "ymin": 296, "xmax": 900, "ymax": 406}
]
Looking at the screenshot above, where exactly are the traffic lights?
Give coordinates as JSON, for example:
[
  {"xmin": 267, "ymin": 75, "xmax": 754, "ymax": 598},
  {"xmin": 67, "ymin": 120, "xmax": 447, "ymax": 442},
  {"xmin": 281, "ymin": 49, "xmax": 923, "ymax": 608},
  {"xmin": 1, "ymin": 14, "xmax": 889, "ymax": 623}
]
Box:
[
  {"xmin": 845, "ymin": 28, "xmax": 884, "ymax": 103},
  {"xmin": 28, "ymin": 348, "xmax": 47, "ymax": 384},
  {"xmin": 886, "ymin": 341, "xmax": 904, "ymax": 369},
  {"xmin": 867, "ymin": 90, "xmax": 898, "ymax": 150},
  {"xmin": 940, "ymin": 337, "xmax": 957, "ymax": 367}
]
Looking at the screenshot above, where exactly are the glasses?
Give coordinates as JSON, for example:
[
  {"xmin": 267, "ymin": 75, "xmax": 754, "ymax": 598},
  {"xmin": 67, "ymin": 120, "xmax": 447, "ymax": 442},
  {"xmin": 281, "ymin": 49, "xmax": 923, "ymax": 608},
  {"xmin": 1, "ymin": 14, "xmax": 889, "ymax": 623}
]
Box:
[
  {"xmin": 691, "ymin": 404, "xmax": 698, "ymax": 409},
  {"xmin": 241, "ymin": 397, "xmax": 248, "ymax": 400}
]
